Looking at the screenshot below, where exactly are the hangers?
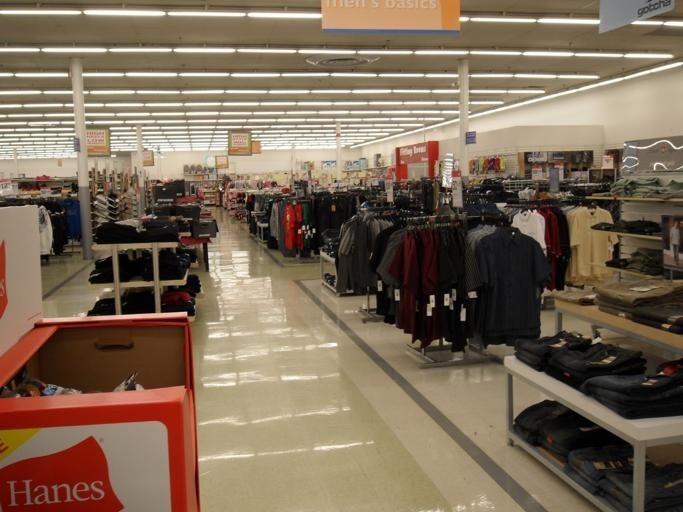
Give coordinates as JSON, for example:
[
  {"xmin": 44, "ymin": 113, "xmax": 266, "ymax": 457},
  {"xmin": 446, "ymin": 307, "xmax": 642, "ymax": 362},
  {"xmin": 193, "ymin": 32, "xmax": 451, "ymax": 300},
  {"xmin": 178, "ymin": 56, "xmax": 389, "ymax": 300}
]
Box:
[{"xmin": 1, "ymin": 196, "xmax": 66, "ymax": 213}]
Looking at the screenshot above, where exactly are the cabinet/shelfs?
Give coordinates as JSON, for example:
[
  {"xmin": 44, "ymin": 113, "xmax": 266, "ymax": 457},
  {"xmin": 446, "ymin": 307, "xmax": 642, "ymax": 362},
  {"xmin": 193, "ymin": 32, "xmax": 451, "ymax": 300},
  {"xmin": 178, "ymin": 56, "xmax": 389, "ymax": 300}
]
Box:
[
  {"xmin": 588, "ymin": 195, "xmax": 683, "ymax": 281},
  {"xmin": 505, "ymin": 281, "xmax": 683, "ymax": 511},
  {"xmin": 91, "ymin": 241, "xmax": 197, "ymax": 321},
  {"xmin": 89, "ymin": 168, "xmax": 141, "ymax": 232},
  {"xmin": 8, "ymin": 175, "xmax": 79, "ymax": 194},
  {"xmin": 203, "ymin": 189, "xmax": 217, "ymax": 205}
]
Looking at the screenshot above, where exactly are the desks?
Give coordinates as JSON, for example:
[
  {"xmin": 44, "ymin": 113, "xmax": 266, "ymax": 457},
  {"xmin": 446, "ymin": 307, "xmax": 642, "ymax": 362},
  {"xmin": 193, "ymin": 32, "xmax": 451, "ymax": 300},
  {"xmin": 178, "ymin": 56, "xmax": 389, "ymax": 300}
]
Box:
[
  {"xmin": 320, "ymin": 249, "xmax": 378, "ymax": 297},
  {"xmin": 182, "ymin": 237, "xmax": 211, "ymax": 271}
]
